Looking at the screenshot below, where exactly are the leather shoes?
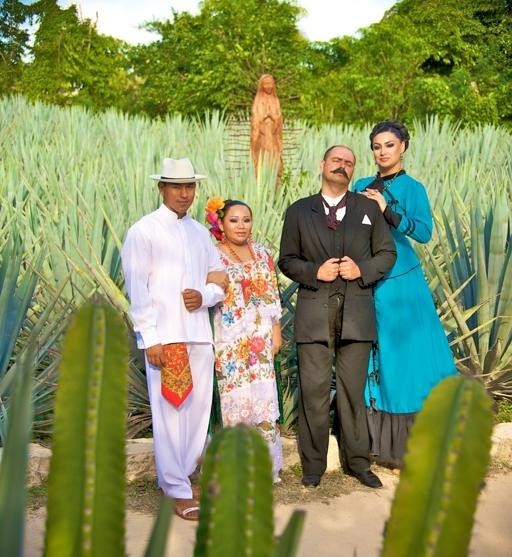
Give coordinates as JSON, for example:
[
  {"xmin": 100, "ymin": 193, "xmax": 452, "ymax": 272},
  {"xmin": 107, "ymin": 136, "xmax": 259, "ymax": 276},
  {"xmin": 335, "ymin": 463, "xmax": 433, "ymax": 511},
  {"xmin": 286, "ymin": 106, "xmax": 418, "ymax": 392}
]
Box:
[
  {"xmin": 344, "ymin": 468, "xmax": 383, "ymax": 487},
  {"xmin": 301, "ymin": 474, "xmax": 321, "ymax": 486}
]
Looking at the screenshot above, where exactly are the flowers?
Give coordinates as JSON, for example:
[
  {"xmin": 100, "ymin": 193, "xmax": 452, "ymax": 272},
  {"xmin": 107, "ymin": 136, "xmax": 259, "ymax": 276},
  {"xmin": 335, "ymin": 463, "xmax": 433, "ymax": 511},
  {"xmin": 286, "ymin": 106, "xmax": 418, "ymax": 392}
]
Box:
[{"xmin": 205, "ymin": 195, "xmax": 225, "ymax": 241}]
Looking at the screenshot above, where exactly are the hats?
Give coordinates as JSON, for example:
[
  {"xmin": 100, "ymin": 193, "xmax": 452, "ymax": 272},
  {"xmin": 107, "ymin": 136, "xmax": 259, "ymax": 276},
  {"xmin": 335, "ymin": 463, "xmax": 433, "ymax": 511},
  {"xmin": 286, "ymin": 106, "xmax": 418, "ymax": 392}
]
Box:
[{"xmin": 148, "ymin": 157, "xmax": 208, "ymax": 184}]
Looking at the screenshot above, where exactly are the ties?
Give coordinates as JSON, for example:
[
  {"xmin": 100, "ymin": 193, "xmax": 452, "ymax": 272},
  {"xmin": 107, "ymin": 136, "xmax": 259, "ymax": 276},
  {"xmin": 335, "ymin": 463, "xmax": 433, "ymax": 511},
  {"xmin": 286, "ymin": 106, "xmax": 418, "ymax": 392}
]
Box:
[{"xmin": 322, "ymin": 191, "xmax": 347, "ymax": 230}]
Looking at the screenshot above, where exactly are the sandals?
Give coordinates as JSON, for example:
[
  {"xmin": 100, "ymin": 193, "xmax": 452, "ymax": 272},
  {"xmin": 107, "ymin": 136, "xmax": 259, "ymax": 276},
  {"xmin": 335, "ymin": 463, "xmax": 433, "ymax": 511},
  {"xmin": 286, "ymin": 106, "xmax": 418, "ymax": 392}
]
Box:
[{"xmin": 173, "ymin": 501, "xmax": 199, "ymax": 520}]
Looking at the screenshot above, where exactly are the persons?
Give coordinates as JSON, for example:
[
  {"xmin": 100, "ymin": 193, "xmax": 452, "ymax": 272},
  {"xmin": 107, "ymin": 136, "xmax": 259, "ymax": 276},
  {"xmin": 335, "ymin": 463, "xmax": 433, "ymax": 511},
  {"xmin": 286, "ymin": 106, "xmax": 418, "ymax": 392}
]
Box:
[
  {"xmin": 207, "ymin": 199, "xmax": 286, "ymax": 486},
  {"xmin": 351, "ymin": 120, "xmax": 462, "ymax": 470},
  {"xmin": 250, "ymin": 74, "xmax": 283, "ymax": 185},
  {"xmin": 120, "ymin": 156, "xmax": 228, "ymax": 522},
  {"xmin": 277, "ymin": 145, "xmax": 399, "ymax": 488}
]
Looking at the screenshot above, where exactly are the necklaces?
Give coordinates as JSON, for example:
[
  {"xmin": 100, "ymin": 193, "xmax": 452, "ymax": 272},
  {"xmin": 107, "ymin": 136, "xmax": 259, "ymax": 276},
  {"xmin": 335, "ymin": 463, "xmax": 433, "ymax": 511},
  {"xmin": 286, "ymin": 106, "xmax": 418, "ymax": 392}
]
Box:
[
  {"xmin": 379, "ymin": 168, "xmax": 402, "ymax": 191},
  {"xmin": 222, "ymin": 236, "xmax": 256, "ymax": 262}
]
